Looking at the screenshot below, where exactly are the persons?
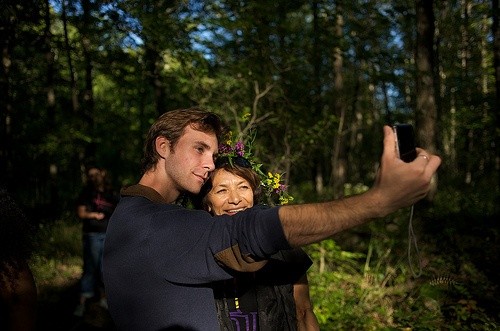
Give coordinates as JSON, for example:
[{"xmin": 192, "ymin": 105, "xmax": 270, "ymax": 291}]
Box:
[
  {"xmin": 72, "ymin": 162, "xmax": 120, "ymax": 319},
  {"xmin": 191, "ymin": 152, "xmax": 322, "ymax": 331},
  {"xmin": 102, "ymin": 108, "xmax": 441, "ymax": 331}
]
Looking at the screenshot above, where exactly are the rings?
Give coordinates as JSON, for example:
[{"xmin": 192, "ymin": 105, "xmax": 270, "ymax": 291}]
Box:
[{"xmin": 416, "ymin": 154, "xmax": 430, "ymax": 163}]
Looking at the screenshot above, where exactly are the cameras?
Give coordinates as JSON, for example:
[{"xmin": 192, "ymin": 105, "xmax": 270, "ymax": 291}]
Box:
[{"xmin": 391, "ymin": 123, "xmax": 417, "ymax": 164}]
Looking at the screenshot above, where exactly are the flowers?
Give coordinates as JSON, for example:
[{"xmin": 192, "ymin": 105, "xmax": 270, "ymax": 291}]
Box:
[
  {"xmin": 263, "ymin": 172, "xmax": 295, "ymax": 206},
  {"xmin": 218, "ymin": 134, "xmax": 247, "ymax": 160}
]
